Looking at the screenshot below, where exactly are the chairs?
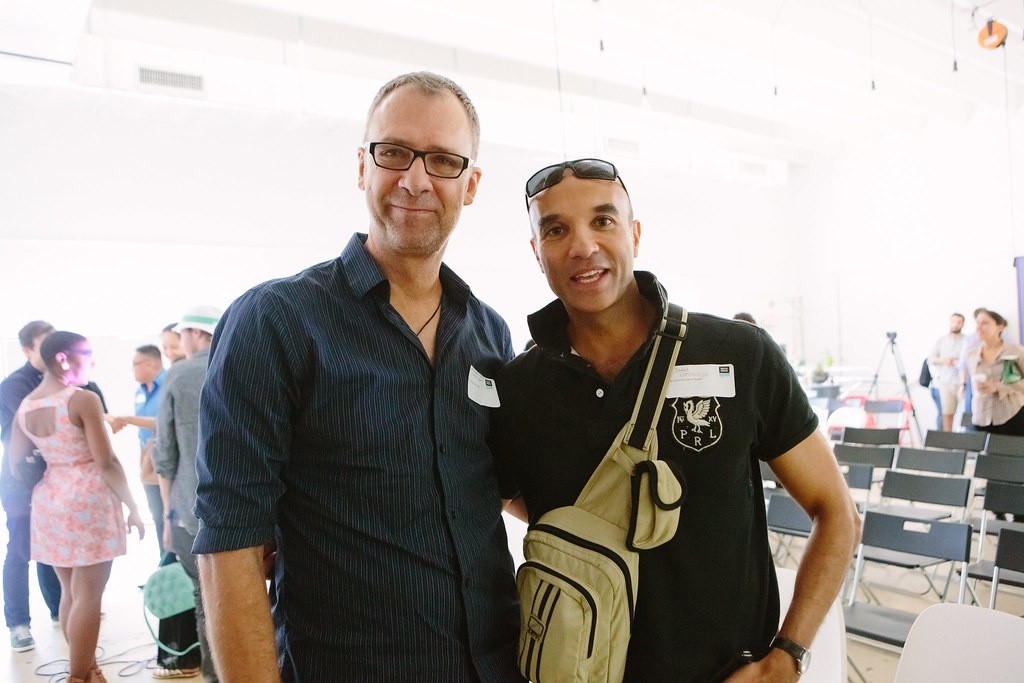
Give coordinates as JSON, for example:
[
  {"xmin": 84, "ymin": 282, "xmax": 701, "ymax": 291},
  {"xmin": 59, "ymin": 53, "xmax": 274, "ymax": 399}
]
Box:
[
  {"xmin": 892, "ymin": 602, "xmax": 1024, "ymax": 683},
  {"xmin": 758, "ymin": 384, "xmax": 1024, "ymax": 647}
]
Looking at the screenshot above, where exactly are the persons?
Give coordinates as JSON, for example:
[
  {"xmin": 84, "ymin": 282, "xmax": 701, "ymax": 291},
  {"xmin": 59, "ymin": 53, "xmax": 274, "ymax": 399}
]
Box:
[
  {"xmin": 925, "ymin": 306, "xmax": 1024, "ymax": 437},
  {"xmin": 189, "ymin": 71, "xmax": 532, "ymax": 683},
  {"xmin": 498, "ymin": 157, "xmax": 864, "ymax": 683},
  {"xmin": 0, "ymin": 303, "xmax": 277, "ymax": 683},
  {"xmin": 731, "ymin": 312, "xmax": 756, "ymax": 326}
]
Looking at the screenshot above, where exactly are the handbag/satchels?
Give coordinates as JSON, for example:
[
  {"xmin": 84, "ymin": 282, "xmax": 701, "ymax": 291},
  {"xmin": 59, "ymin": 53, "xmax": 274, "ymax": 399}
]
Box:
[
  {"xmin": 16, "ymin": 450, "xmax": 45, "ymax": 489},
  {"xmin": 517, "ymin": 304, "xmax": 689, "ymax": 683},
  {"xmin": 1001, "ymin": 359, "xmax": 1023, "ymax": 385},
  {"xmin": 140, "ymin": 550, "xmax": 196, "ymax": 621}
]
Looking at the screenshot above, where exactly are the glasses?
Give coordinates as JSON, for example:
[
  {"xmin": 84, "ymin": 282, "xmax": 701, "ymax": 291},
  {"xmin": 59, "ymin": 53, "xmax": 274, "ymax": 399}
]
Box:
[
  {"xmin": 361, "ymin": 143, "xmax": 470, "ymax": 178},
  {"xmin": 523, "ymin": 158, "xmax": 629, "ymax": 212}
]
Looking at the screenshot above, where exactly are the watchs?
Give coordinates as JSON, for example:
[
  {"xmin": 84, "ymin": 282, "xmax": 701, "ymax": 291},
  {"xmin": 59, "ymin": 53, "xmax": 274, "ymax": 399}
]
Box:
[{"xmin": 769, "ymin": 635, "xmax": 812, "ymax": 676}]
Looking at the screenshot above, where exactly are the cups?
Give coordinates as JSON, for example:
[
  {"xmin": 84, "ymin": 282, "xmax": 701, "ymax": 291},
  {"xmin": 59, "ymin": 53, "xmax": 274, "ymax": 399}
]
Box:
[{"xmin": 972, "ymin": 373, "xmax": 987, "ymax": 396}]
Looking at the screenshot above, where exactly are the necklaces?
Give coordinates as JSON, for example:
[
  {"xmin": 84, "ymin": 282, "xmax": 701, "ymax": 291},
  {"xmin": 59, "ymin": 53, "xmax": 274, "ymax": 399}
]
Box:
[{"xmin": 414, "ymin": 286, "xmax": 445, "ymax": 338}]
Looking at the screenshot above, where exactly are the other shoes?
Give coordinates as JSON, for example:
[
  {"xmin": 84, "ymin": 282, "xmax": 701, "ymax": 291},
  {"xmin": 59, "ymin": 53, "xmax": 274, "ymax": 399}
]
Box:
[{"xmin": 9, "ymin": 626, "xmax": 37, "ymax": 651}]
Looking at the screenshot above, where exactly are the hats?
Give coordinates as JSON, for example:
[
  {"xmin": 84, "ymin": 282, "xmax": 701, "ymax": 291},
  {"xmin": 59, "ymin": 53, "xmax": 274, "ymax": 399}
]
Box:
[{"xmin": 172, "ymin": 304, "xmax": 220, "ymax": 335}]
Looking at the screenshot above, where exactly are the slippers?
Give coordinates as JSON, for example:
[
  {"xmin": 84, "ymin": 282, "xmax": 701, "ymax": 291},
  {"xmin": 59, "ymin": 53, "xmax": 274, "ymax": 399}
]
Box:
[{"xmin": 152, "ymin": 667, "xmax": 200, "ymax": 679}]
